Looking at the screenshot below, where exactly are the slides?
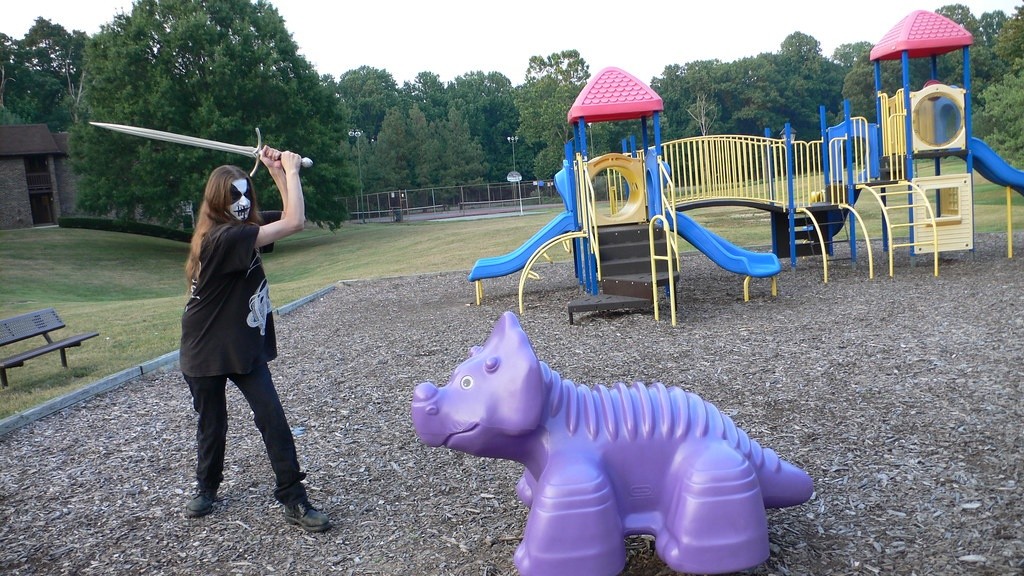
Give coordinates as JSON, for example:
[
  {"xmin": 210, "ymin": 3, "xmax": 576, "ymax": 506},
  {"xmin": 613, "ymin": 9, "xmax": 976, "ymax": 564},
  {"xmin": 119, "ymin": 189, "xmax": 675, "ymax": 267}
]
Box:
[
  {"xmin": 660, "ymin": 210, "xmax": 781, "ymax": 277},
  {"xmin": 956, "ymin": 136, "xmax": 1024, "ymax": 195},
  {"xmin": 466, "ymin": 212, "xmax": 575, "ymax": 282}
]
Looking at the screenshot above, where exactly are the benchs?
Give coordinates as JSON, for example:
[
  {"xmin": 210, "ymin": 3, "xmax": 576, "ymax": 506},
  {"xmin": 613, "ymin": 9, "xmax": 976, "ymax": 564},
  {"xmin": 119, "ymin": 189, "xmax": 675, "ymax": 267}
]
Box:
[{"xmin": 0, "ymin": 308, "xmax": 100, "ymax": 387}]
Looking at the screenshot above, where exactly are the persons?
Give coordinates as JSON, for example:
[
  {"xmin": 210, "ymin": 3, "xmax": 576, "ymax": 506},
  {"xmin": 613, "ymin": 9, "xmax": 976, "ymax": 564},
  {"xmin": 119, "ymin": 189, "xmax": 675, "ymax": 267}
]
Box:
[{"xmin": 179, "ymin": 145, "xmax": 330, "ymax": 534}]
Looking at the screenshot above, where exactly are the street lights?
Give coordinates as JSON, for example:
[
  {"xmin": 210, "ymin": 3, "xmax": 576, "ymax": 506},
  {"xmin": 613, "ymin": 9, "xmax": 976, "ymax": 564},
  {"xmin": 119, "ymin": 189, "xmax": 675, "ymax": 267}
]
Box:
[
  {"xmin": 347, "ymin": 128, "xmax": 365, "ymax": 224},
  {"xmin": 507, "ymin": 136, "xmax": 518, "ymax": 206}
]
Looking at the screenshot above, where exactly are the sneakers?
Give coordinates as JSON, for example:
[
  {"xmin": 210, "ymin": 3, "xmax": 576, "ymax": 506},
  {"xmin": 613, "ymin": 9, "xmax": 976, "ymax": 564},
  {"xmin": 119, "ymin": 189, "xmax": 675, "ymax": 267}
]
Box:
[
  {"xmin": 187, "ymin": 487, "xmax": 217, "ymax": 515},
  {"xmin": 285, "ymin": 500, "xmax": 331, "ymax": 532}
]
